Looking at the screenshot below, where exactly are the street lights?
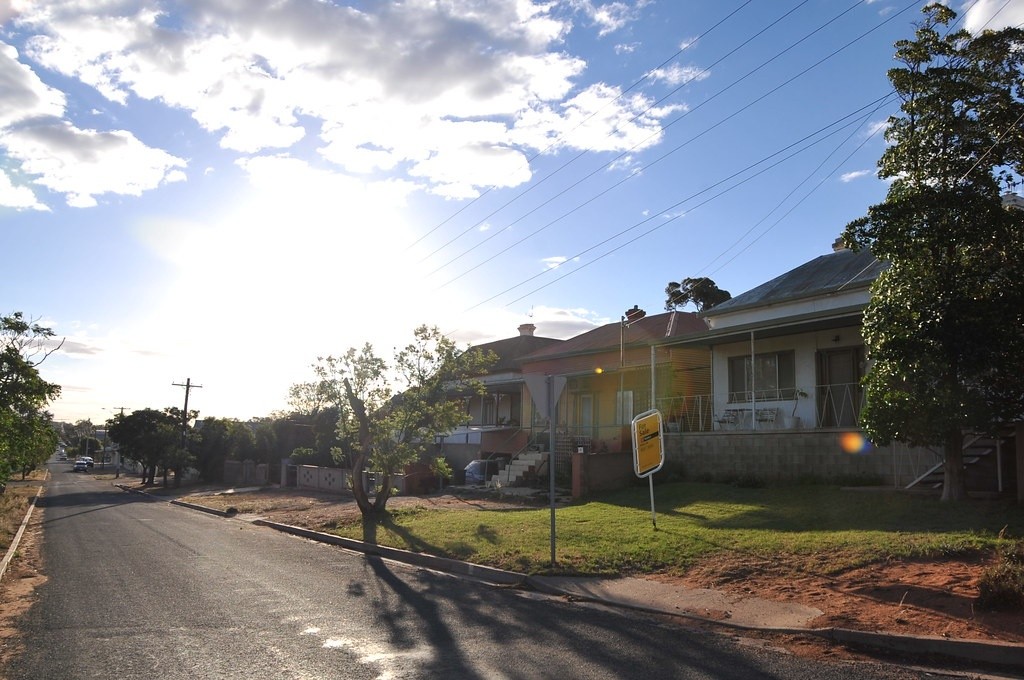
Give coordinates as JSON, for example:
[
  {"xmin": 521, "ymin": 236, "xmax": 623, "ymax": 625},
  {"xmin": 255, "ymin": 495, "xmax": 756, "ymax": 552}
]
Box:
[{"xmin": 103, "ymin": 407, "xmax": 119, "ymax": 418}]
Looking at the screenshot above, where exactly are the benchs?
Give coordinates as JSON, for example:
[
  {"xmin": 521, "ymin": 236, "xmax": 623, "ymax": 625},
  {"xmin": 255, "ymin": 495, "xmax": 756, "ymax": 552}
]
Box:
[{"xmin": 714, "ymin": 408, "xmax": 778, "ymax": 432}]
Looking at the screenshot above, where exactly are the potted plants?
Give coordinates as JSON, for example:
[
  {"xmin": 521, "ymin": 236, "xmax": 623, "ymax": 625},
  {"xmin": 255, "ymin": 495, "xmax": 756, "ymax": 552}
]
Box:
[
  {"xmin": 668, "ymin": 415, "xmax": 682, "ymax": 434},
  {"xmin": 782, "ymin": 390, "xmax": 808, "ymax": 429}
]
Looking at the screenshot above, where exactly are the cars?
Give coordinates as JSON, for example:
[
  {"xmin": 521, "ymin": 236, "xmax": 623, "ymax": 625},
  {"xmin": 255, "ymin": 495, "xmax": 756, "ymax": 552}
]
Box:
[
  {"xmin": 74, "ymin": 460, "xmax": 87, "ymax": 471},
  {"xmin": 81, "ymin": 457, "xmax": 94, "ymax": 468}
]
